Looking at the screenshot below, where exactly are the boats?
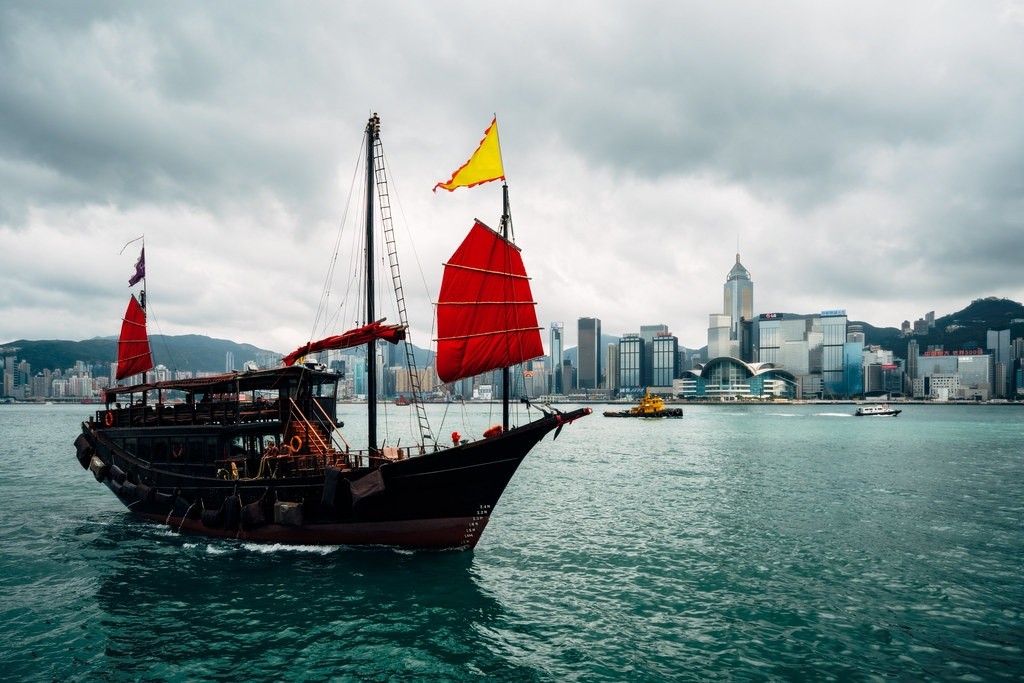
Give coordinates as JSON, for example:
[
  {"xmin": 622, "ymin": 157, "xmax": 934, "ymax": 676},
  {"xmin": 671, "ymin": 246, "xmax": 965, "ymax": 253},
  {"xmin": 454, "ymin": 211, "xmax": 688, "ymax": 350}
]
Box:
[
  {"xmin": 394, "ymin": 395, "xmax": 411, "ymax": 407},
  {"xmin": 603, "ymin": 386, "xmax": 684, "ymax": 420},
  {"xmin": 855, "ymin": 405, "xmax": 903, "ymax": 418}
]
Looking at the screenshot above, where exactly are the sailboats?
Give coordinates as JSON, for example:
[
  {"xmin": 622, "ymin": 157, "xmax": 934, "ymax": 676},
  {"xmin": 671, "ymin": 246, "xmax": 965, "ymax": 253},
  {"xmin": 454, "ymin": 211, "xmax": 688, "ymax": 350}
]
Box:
[{"xmin": 72, "ymin": 111, "xmax": 593, "ymax": 559}]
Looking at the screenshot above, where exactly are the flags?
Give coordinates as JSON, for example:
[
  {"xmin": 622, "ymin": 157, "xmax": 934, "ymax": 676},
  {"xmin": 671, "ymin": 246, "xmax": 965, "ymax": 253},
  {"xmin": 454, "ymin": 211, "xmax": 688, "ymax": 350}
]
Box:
[{"xmin": 128, "ymin": 248, "xmax": 145, "ymax": 287}]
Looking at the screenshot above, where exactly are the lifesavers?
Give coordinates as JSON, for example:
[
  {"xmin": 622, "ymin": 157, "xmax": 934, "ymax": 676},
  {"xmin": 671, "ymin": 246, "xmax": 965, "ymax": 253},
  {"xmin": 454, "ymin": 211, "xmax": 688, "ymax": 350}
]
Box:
[
  {"xmin": 105, "ymin": 412, "xmax": 114, "ymax": 427},
  {"xmin": 289, "ymin": 436, "xmax": 303, "ymax": 453}
]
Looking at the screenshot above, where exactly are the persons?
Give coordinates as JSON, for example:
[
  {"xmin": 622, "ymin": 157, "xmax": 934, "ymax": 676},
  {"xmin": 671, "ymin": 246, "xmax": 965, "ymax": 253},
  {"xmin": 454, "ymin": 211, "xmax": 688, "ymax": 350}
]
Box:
[
  {"xmin": 263, "ymin": 441, "xmax": 294, "ymax": 477},
  {"xmin": 172, "ymin": 441, "xmax": 183, "ymax": 463},
  {"xmin": 253, "ymin": 396, "xmax": 279, "ymax": 410},
  {"xmin": 201, "ymin": 394, "xmax": 212, "ymax": 402},
  {"xmin": 115, "ymin": 403, "xmax": 122, "ymax": 410}
]
